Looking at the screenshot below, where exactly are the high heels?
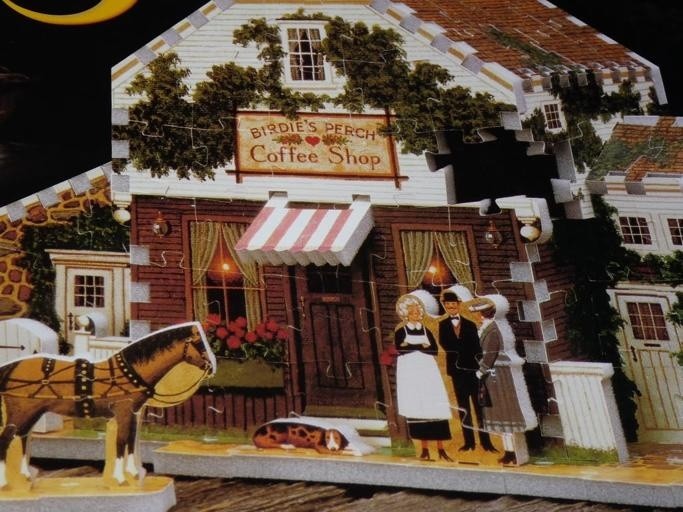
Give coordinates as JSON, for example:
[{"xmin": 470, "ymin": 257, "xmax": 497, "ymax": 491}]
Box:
[
  {"xmin": 498, "ymin": 450, "xmax": 515, "ymax": 465},
  {"xmin": 437, "ymin": 447, "xmax": 452, "ymax": 460},
  {"xmin": 418, "ymin": 447, "xmax": 430, "ymax": 460}
]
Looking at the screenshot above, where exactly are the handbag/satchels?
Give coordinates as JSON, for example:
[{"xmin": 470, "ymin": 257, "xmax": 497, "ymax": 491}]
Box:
[{"xmin": 477, "ymin": 379, "xmax": 491, "ymax": 408}]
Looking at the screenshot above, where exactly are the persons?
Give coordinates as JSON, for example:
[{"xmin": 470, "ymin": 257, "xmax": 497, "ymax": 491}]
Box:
[
  {"xmin": 467, "ymin": 303, "xmax": 527, "ymax": 465},
  {"xmin": 437, "ymin": 293, "xmax": 499, "ymax": 454},
  {"xmin": 395, "ymin": 297, "xmax": 453, "ymax": 464}
]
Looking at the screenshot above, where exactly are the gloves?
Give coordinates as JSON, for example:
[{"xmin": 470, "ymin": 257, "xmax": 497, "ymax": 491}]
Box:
[{"xmin": 475, "ymin": 371, "xmax": 483, "ymax": 379}]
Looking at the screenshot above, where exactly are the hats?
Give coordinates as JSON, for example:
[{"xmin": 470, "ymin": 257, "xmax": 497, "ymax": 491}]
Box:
[
  {"xmin": 441, "ymin": 292, "xmax": 461, "ymax": 303},
  {"xmin": 468, "ymin": 302, "xmax": 493, "ymax": 312}
]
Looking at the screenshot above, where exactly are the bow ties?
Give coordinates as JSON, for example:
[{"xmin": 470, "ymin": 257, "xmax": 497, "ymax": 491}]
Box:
[{"xmin": 450, "ymin": 317, "xmax": 458, "ymax": 320}]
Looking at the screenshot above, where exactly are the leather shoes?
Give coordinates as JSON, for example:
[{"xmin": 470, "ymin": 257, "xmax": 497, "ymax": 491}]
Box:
[
  {"xmin": 482, "ymin": 443, "xmax": 501, "ymax": 453},
  {"xmin": 459, "ymin": 442, "xmax": 477, "ymax": 452}
]
